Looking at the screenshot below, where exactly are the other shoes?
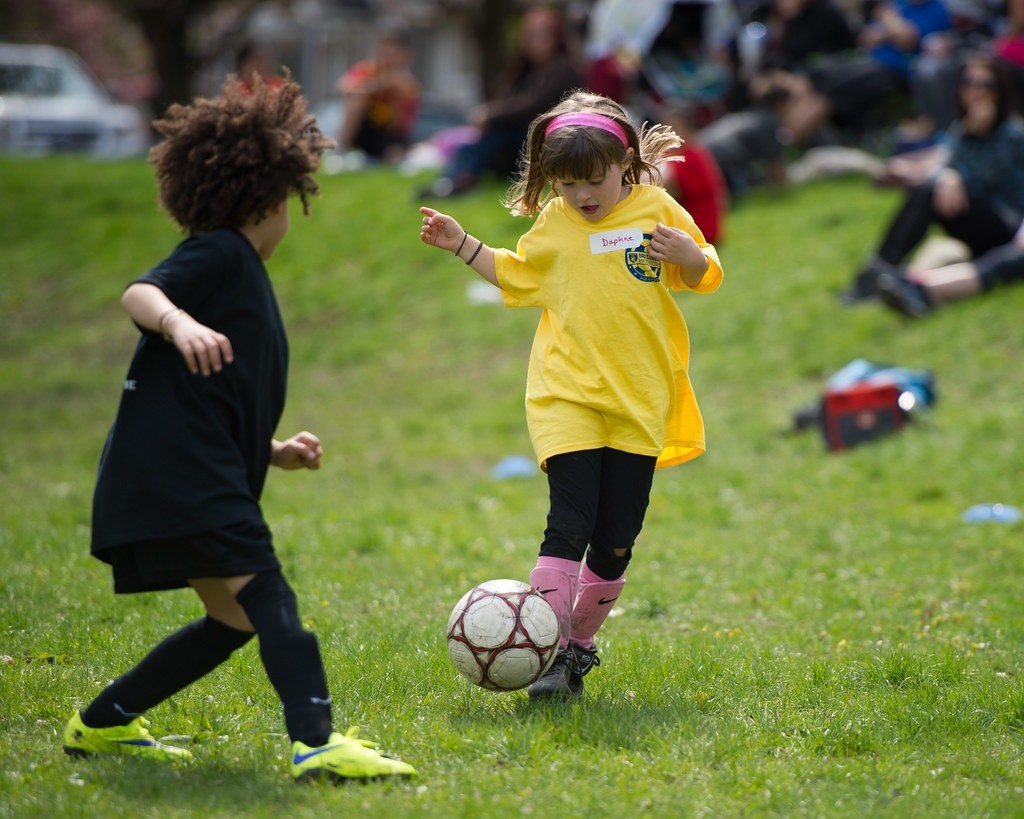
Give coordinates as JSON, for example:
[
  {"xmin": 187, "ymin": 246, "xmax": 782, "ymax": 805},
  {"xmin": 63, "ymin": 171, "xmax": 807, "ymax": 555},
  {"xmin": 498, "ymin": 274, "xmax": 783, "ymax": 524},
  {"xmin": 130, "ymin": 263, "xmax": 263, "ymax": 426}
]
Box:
[
  {"xmin": 831, "ymin": 276, "xmax": 883, "ymax": 301},
  {"xmin": 871, "ymin": 266, "xmax": 934, "ymax": 319}
]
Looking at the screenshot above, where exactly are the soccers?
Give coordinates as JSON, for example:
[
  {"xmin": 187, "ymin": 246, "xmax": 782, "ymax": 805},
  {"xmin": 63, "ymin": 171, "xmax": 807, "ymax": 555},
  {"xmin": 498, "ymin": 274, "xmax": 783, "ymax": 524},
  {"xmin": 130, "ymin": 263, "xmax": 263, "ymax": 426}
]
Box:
[{"xmin": 446, "ymin": 575, "xmax": 560, "ymax": 695}]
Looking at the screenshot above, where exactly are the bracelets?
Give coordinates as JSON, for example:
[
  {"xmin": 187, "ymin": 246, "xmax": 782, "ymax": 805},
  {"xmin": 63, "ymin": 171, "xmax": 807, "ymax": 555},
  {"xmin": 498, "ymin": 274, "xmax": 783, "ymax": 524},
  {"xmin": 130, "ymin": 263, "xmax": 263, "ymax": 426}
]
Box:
[
  {"xmin": 454, "ymin": 230, "xmax": 483, "ymax": 265},
  {"xmin": 160, "ymin": 307, "xmax": 183, "ymax": 333}
]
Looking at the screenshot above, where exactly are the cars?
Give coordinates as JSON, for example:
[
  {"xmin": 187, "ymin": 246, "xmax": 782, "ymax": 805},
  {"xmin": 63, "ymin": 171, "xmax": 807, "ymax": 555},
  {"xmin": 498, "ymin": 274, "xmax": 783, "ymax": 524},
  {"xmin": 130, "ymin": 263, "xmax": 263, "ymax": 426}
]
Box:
[{"xmin": 0, "ymin": 43, "xmax": 147, "ymax": 162}]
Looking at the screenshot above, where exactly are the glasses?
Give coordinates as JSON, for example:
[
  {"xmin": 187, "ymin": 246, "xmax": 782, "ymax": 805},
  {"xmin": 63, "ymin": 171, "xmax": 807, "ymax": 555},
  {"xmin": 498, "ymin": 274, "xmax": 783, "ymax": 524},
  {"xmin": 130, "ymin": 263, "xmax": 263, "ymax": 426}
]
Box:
[{"xmin": 960, "ymin": 77, "xmax": 988, "ymax": 91}]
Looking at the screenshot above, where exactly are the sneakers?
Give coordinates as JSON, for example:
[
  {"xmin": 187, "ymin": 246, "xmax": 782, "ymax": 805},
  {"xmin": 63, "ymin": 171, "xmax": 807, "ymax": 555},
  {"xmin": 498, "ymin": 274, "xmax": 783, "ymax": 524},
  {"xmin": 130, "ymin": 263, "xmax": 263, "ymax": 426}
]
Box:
[
  {"xmin": 64, "ymin": 709, "xmax": 197, "ymax": 768},
  {"xmin": 529, "ymin": 641, "xmax": 602, "ymax": 702},
  {"xmin": 292, "ymin": 725, "xmax": 418, "ymax": 784}
]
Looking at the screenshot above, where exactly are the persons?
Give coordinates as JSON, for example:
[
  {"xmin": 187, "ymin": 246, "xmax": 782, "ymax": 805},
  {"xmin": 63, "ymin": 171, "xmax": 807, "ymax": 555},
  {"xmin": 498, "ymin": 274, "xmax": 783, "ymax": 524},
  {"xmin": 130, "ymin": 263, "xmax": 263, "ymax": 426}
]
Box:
[
  {"xmin": 418, "ymin": 88, "xmax": 723, "ymax": 702},
  {"xmin": 64, "ymin": 70, "xmax": 419, "ymax": 782},
  {"xmin": 1, "ymin": 0, "xmax": 1024, "ymax": 321}
]
